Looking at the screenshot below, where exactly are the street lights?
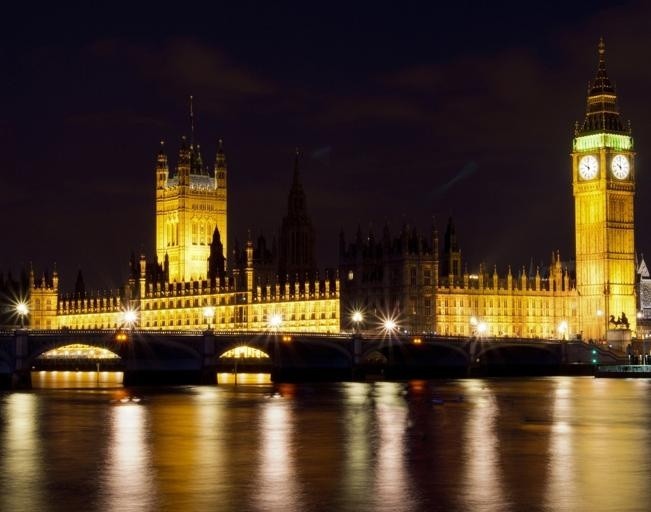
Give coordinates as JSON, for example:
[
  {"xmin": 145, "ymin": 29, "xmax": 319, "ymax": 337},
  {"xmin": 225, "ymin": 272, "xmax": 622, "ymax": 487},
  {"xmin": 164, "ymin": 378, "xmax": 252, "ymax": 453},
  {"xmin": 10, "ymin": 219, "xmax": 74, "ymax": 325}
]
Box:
[
  {"xmin": 204, "ymin": 308, "xmax": 213, "ymax": 329},
  {"xmin": 352, "ymin": 312, "xmax": 363, "ymax": 333},
  {"xmin": 561, "ymin": 321, "xmax": 568, "ymax": 340},
  {"xmin": 16, "ymin": 303, "xmax": 28, "ymax": 328}
]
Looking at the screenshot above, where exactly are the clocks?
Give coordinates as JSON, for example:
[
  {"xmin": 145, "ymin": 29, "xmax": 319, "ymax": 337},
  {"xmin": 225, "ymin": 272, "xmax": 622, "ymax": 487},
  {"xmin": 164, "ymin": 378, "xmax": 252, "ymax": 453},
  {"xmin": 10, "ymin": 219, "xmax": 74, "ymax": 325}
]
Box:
[
  {"xmin": 609, "ymin": 152, "xmax": 631, "ymax": 179},
  {"xmin": 578, "ymin": 155, "xmax": 600, "ymax": 179}
]
